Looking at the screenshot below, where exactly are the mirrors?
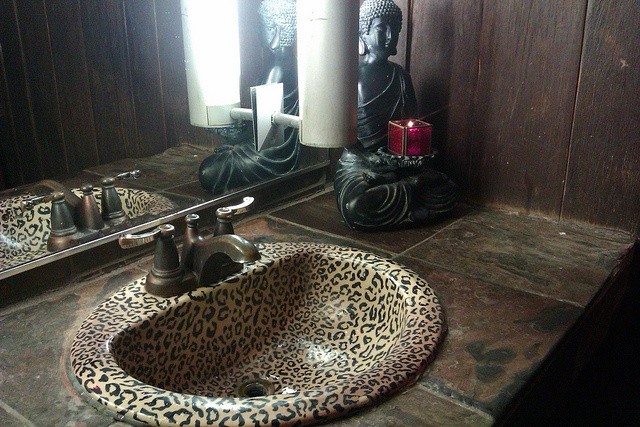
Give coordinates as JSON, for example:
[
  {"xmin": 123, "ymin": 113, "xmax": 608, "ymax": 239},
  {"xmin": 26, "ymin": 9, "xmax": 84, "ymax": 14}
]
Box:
[{"xmin": 0, "ymin": 0, "xmax": 329, "ymax": 278}]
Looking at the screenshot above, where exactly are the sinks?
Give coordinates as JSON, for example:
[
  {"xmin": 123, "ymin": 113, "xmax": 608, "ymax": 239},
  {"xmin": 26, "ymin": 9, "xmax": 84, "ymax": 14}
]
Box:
[
  {"xmin": 69, "ymin": 241, "xmax": 446, "ymax": 426},
  {"xmin": 0, "ymin": 188, "xmax": 176, "ymax": 269}
]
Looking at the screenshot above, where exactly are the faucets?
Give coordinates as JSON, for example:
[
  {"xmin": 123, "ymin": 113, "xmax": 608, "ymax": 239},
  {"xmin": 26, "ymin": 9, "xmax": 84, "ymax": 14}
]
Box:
[
  {"xmin": 17, "ymin": 179, "xmax": 105, "ymax": 239},
  {"xmin": 181, "ymin": 212, "xmax": 261, "ymax": 289}
]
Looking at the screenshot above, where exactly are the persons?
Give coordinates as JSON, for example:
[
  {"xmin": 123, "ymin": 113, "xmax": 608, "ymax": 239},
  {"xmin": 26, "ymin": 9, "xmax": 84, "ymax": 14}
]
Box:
[
  {"xmin": 334, "ymin": 1, "xmax": 458, "ymax": 230},
  {"xmin": 199, "ymin": 0, "xmax": 297, "ymax": 193}
]
